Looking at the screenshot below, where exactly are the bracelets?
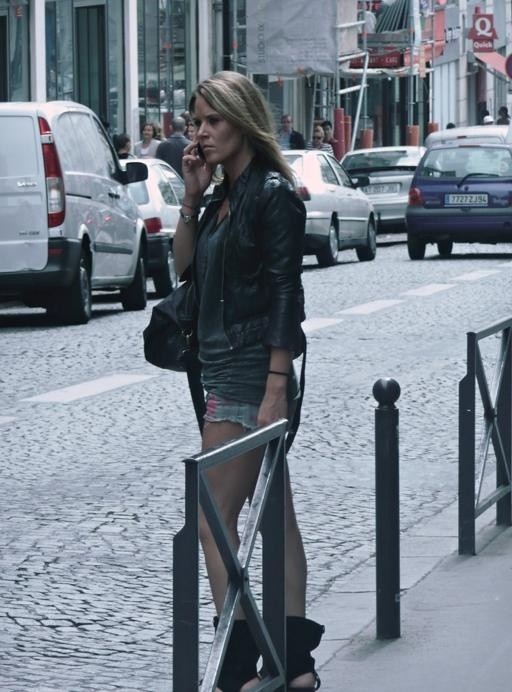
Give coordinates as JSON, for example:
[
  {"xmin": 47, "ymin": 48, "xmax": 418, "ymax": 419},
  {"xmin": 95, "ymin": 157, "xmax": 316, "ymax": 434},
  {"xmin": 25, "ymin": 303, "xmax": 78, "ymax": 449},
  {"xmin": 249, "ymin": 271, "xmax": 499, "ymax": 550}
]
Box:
[
  {"xmin": 266, "ymin": 368, "xmax": 290, "ymax": 379},
  {"xmin": 178, "ymin": 203, "xmax": 202, "ymax": 212},
  {"xmin": 181, "ymin": 213, "xmax": 196, "ymax": 223}
]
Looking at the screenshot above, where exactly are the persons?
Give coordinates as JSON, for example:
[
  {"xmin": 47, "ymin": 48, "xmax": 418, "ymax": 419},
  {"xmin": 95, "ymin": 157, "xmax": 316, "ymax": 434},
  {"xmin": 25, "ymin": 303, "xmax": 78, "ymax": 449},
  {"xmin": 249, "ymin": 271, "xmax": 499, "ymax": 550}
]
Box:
[
  {"xmin": 101, "ymin": 104, "xmax": 339, "ymax": 165},
  {"xmin": 171, "ymin": 71, "xmax": 321, "ymax": 690},
  {"xmin": 495, "ymin": 107, "xmax": 510, "ymax": 126},
  {"xmin": 481, "ymin": 110, "xmax": 494, "ymax": 127},
  {"xmin": 446, "ymin": 123, "xmax": 456, "ymax": 130}
]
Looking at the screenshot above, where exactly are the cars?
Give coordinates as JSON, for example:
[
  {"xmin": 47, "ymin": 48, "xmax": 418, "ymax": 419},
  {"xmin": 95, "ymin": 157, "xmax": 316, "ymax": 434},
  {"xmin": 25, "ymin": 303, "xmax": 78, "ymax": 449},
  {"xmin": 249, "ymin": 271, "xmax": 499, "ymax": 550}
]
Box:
[{"xmin": 73, "ymin": 79, "xmax": 187, "ymax": 132}]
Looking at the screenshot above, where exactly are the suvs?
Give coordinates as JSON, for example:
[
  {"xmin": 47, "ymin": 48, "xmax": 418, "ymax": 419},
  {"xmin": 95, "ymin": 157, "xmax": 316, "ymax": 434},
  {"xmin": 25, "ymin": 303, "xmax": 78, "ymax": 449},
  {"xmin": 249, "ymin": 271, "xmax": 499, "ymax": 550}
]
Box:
[{"xmin": 119, "ymin": 154, "xmax": 193, "ymax": 294}]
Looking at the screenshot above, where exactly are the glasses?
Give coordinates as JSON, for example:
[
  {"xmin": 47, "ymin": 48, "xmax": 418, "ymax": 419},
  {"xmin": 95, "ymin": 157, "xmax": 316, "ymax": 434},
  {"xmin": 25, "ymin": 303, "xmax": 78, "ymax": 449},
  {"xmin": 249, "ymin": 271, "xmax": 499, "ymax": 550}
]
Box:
[{"xmin": 311, "ymin": 135, "xmax": 324, "ymax": 140}]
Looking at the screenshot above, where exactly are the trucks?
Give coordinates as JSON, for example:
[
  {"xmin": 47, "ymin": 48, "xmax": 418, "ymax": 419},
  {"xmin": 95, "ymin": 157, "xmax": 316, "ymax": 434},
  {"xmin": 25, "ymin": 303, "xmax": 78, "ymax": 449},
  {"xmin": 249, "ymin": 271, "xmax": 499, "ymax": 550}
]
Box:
[{"xmin": 0, "ymin": 97, "xmax": 152, "ymax": 320}]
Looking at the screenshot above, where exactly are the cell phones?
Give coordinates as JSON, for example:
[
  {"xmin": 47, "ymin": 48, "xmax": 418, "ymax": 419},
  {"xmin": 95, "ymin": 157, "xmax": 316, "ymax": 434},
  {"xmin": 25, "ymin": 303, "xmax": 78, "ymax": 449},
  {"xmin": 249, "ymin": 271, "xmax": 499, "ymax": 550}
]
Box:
[{"xmin": 196, "ymin": 142, "xmax": 205, "ymax": 162}]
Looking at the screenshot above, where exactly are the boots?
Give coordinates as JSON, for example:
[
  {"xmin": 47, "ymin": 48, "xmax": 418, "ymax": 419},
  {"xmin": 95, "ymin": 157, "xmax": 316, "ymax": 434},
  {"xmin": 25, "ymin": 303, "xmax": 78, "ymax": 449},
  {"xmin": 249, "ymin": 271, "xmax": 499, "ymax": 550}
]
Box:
[
  {"xmin": 211, "ymin": 616, "xmax": 262, "ymax": 692},
  {"xmin": 258, "ymin": 614, "xmax": 321, "ymax": 692}
]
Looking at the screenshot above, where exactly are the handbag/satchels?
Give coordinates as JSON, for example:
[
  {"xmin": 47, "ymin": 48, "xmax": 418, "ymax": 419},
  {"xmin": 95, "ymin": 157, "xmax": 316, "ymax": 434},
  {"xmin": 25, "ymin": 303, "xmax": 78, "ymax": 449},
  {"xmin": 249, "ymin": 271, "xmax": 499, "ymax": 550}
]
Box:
[{"xmin": 140, "ymin": 278, "xmax": 200, "ymax": 376}]
